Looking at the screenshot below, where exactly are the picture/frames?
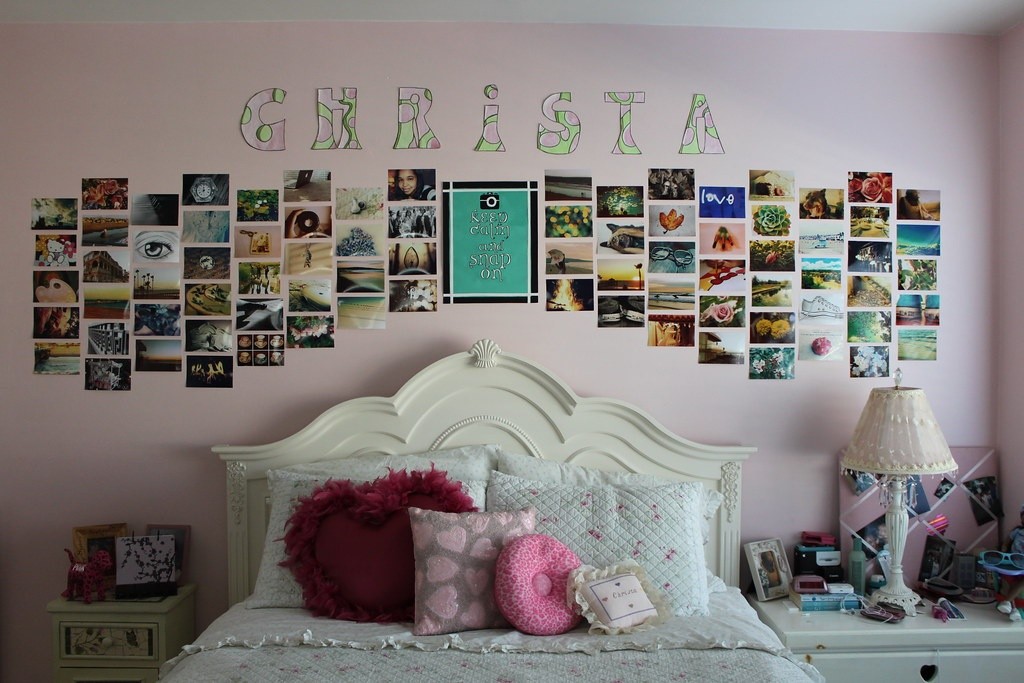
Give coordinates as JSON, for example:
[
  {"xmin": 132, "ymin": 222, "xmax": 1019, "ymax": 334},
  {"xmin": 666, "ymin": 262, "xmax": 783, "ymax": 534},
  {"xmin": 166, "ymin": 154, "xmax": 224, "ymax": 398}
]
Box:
[
  {"xmin": 744, "ymin": 537, "xmax": 793, "ymax": 602},
  {"xmin": 146, "ymin": 524, "xmax": 192, "ymax": 561},
  {"xmin": 72, "ymin": 522, "xmax": 127, "ymax": 576}
]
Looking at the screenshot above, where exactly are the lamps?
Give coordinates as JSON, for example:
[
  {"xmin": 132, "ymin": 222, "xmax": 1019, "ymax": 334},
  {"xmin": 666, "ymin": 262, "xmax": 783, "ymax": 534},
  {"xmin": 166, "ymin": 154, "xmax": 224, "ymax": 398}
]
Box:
[{"xmin": 840, "ymin": 367, "xmax": 959, "ymax": 615}]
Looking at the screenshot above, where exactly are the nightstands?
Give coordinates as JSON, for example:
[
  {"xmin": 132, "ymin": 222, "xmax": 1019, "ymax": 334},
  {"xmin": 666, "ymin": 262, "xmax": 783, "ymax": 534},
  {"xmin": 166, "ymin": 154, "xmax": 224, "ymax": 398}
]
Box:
[
  {"xmin": 45, "ymin": 581, "xmax": 197, "ymax": 683},
  {"xmin": 750, "ymin": 598, "xmax": 1024, "ymax": 683}
]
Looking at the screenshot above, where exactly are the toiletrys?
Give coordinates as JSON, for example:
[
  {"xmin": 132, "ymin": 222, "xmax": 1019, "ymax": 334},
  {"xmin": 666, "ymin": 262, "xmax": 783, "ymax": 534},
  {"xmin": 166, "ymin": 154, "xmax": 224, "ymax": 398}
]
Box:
[{"xmin": 848, "ymin": 538, "xmax": 867, "ymax": 594}]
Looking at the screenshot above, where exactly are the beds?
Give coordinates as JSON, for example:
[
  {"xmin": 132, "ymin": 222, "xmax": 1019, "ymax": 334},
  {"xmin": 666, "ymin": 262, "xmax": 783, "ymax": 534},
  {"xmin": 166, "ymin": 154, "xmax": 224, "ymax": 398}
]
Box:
[{"xmin": 159, "ymin": 338, "xmax": 827, "ymax": 683}]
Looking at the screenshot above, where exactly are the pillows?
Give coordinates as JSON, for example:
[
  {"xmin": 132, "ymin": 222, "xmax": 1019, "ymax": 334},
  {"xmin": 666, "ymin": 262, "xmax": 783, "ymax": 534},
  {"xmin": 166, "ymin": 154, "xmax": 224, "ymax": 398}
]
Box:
[
  {"xmin": 486, "ymin": 470, "xmax": 711, "ymax": 619},
  {"xmin": 408, "ymin": 506, "xmax": 535, "ymax": 636},
  {"xmin": 495, "ymin": 447, "xmax": 728, "ymax": 596},
  {"xmin": 494, "ymin": 534, "xmax": 583, "ymax": 636},
  {"xmin": 246, "ymin": 469, "xmax": 488, "ymax": 610},
  {"xmin": 266, "ymin": 444, "xmax": 500, "ymax": 501},
  {"xmin": 566, "ymin": 561, "xmax": 673, "ymax": 636}
]
